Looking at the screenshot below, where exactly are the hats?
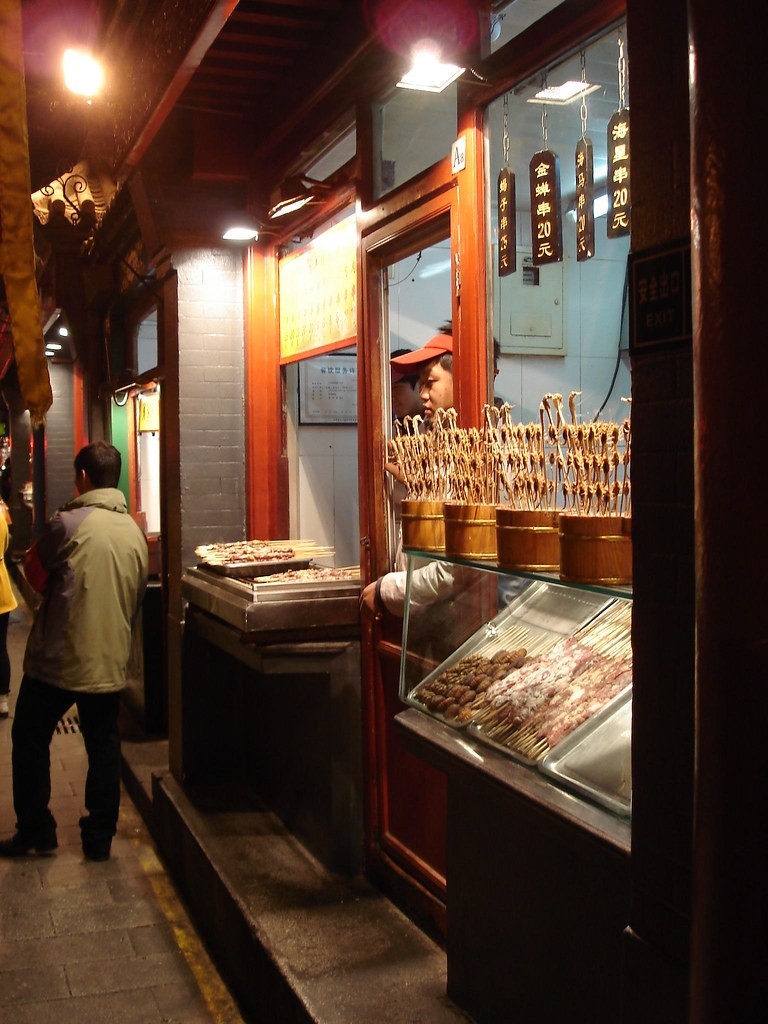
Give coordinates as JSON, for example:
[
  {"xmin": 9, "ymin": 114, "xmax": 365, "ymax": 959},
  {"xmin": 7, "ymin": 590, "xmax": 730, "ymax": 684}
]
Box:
[
  {"xmin": 389, "ymin": 366, "xmax": 403, "ymax": 383},
  {"xmin": 391, "ymin": 334, "xmax": 500, "ymax": 376}
]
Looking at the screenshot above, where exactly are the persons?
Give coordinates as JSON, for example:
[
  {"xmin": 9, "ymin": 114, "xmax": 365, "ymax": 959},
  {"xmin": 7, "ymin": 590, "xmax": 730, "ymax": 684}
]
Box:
[
  {"xmin": 0, "ymin": 479, "xmax": 19, "ymax": 720},
  {"xmin": 0, "ymin": 442, "xmax": 151, "ymax": 859},
  {"xmin": 358, "ymin": 322, "xmax": 509, "ymax": 666}
]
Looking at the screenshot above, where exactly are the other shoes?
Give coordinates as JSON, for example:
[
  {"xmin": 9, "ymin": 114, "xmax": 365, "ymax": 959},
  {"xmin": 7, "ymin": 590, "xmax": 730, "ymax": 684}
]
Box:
[
  {"xmin": 79, "ymin": 816, "xmax": 109, "ymax": 861},
  {"xmin": 0, "ymin": 826, "xmax": 59, "ymax": 858},
  {"xmin": 0, "ymin": 694, "xmax": 9, "ymax": 717}
]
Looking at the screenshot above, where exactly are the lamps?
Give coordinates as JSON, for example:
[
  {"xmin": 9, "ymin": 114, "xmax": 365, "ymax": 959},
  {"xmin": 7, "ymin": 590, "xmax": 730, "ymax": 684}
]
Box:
[
  {"xmin": 527, "ymin": 71, "xmax": 601, "ymax": 105},
  {"xmin": 221, "ymin": 215, "xmax": 284, "ymax": 241},
  {"xmin": 395, "ymin": 39, "xmax": 468, "ymax": 93},
  {"xmin": 269, "ymin": 172, "xmax": 332, "ymax": 219},
  {"xmin": 112, "ymin": 369, "xmax": 143, "ymax": 394}
]
